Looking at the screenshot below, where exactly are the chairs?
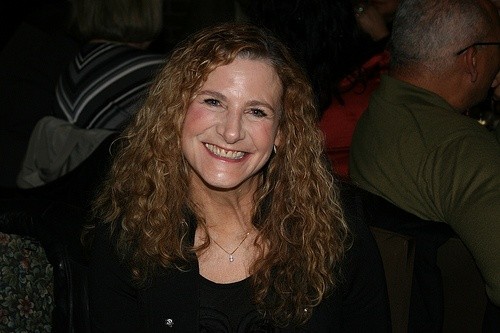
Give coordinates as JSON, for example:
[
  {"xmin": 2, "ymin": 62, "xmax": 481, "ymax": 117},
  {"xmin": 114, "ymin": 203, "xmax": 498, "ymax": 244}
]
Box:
[
  {"xmin": 47, "ymin": 250, "xmax": 75, "ymax": 333},
  {"xmin": 372, "ymin": 229, "xmax": 414, "ymax": 333}
]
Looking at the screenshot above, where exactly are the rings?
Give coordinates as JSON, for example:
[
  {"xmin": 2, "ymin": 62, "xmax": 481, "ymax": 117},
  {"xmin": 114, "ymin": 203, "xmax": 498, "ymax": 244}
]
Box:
[{"xmin": 354, "ymin": 7, "xmax": 368, "ymax": 14}]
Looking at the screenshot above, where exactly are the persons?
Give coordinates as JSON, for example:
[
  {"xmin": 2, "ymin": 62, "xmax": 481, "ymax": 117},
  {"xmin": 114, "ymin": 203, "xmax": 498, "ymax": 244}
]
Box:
[
  {"xmin": 53, "ymin": 0, "xmax": 171, "ymax": 133},
  {"xmin": 79, "ymin": 21, "xmax": 364, "ymax": 332},
  {"xmin": 315, "ymin": 0, "xmax": 496, "ymax": 300}
]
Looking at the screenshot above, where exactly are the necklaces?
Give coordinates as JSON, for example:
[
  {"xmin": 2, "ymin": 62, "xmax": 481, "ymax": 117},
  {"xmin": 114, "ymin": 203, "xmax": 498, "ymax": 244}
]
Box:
[{"xmin": 196, "ymin": 224, "xmax": 256, "ymax": 262}]
